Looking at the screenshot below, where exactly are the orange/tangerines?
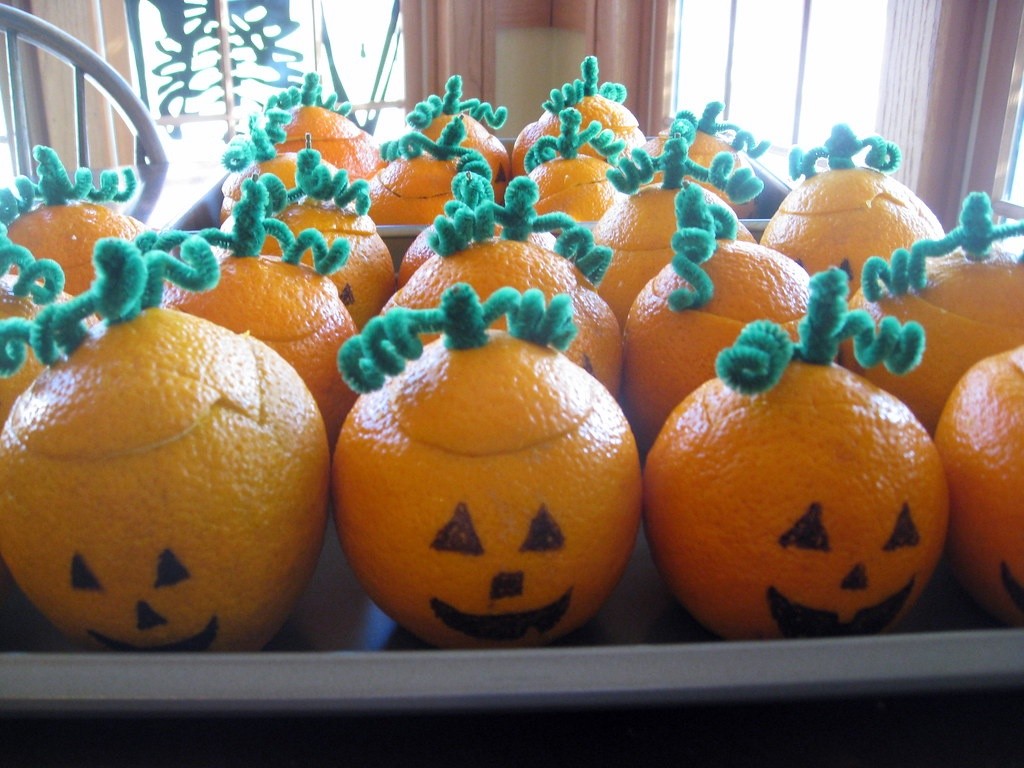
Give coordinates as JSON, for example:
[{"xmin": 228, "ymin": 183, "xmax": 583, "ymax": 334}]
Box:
[{"xmin": 0, "ymin": 93, "xmax": 1024, "ymax": 651}]
